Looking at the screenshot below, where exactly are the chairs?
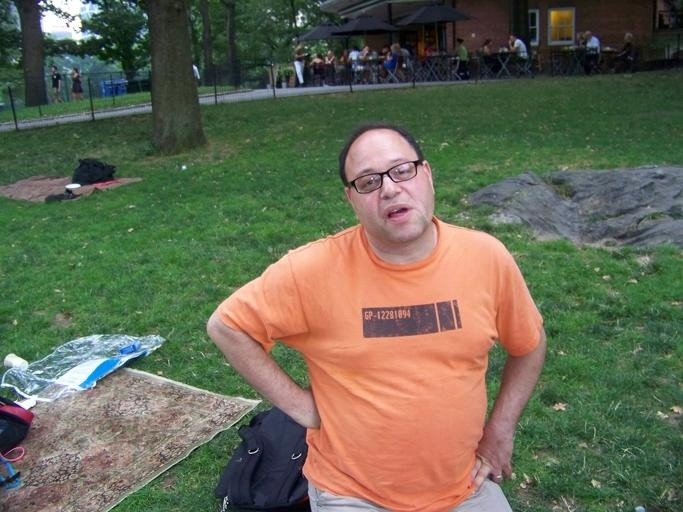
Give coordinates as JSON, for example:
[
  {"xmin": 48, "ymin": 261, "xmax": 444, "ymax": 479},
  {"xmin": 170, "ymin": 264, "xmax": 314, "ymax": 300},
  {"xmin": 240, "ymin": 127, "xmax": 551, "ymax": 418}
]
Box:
[{"xmin": 304, "ymin": 44, "xmax": 619, "ymax": 82}]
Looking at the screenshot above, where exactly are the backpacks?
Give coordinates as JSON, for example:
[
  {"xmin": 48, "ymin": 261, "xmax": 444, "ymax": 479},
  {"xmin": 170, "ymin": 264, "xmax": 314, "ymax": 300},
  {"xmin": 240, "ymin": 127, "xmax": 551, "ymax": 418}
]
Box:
[{"xmin": 214, "ymin": 404, "xmax": 312, "ymax": 512}]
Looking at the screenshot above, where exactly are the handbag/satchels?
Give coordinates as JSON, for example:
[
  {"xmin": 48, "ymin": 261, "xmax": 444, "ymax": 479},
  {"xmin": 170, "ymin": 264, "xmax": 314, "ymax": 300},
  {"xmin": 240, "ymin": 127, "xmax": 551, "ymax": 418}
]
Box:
[{"xmin": 71, "ymin": 157, "xmax": 116, "ymax": 186}]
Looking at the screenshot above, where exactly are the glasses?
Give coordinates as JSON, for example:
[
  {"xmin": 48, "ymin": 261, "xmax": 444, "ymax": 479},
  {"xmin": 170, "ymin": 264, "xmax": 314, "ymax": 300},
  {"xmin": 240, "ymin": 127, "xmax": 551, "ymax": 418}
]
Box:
[{"xmin": 347, "ymin": 159, "xmax": 423, "ymax": 195}]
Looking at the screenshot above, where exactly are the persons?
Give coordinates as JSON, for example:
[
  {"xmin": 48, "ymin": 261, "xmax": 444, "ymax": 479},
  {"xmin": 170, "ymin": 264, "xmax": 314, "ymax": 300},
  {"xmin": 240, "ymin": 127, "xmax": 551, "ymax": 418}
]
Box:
[
  {"xmin": 290, "ymin": 41, "xmax": 413, "ymax": 87},
  {"xmin": 559, "ymin": 31, "xmax": 572, "ymax": 41},
  {"xmin": 577, "ymin": 29, "xmax": 637, "ymax": 75},
  {"xmin": 205, "ymin": 123, "xmax": 547, "ymax": 511},
  {"xmin": 51, "ymin": 65, "xmax": 64, "ymax": 105},
  {"xmin": 421, "ymin": 34, "xmax": 529, "ymax": 81},
  {"xmin": 70, "ymin": 67, "xmax": 84, "ymax": 103}
]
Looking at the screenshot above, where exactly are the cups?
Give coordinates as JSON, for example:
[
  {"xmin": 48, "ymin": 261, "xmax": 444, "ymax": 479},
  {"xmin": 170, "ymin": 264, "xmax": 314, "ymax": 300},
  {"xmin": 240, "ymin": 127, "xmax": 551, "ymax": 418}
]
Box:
[{"xmin": 3, "ymin": 353, "xmax": 28, "ymax": 370}]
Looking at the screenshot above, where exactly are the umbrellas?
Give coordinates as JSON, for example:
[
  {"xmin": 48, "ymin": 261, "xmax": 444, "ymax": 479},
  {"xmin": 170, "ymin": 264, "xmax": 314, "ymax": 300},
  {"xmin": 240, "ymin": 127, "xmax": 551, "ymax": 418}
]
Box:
[{"xmin": 295, "ymin": 3, "xmax": 475, "ymax": 66}]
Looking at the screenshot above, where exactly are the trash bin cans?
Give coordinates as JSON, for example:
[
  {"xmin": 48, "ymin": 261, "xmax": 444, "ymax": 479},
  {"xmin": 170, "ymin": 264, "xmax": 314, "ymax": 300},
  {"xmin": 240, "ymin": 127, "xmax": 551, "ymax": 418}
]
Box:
[{"xmin": 100, "ymin": 79, "xmax": 152, "ymax": 97}]
